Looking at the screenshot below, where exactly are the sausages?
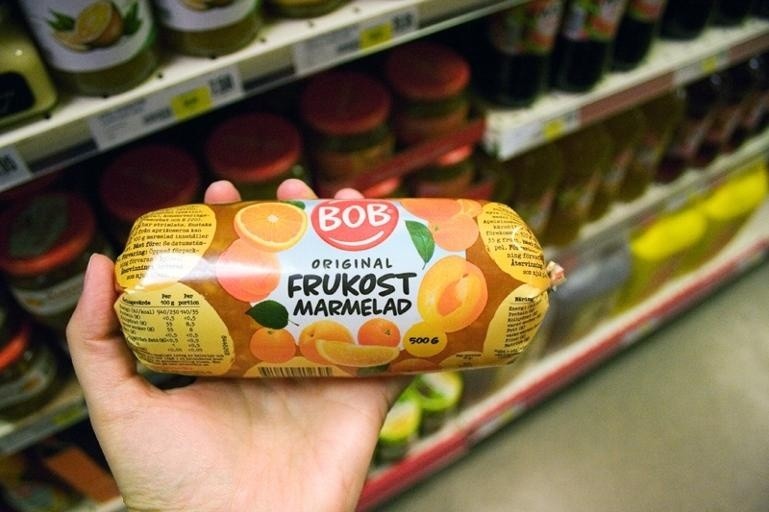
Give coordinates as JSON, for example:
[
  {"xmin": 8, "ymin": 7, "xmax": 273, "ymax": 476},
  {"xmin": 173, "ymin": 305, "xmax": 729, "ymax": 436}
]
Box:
[{"xmin": 114, "ymin": 198, "xmax": 567, "ymax": 380}]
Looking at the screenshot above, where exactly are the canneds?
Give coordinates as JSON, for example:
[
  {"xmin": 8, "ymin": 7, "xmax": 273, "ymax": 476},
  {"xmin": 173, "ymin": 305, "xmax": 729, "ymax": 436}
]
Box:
[{"xmin": 1, "ymin": 1, "xmax": 477, "ymax": 474}]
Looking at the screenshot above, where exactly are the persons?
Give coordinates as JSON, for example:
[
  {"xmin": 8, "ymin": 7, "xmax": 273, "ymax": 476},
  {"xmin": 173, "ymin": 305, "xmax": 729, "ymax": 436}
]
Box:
[{"xmin": 63, "ymin": 177, "xmax": 419, "ymax": 512}]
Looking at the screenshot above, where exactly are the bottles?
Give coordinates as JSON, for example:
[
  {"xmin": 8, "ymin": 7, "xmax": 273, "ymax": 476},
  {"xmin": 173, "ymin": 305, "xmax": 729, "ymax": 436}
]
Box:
[
  {"xmin": 0, "ymin": 0, "xmax": 354, "ymax": 130},
  {"xmin": 474, "ymin": 1, "xmax": 767, "ymax": 245}
]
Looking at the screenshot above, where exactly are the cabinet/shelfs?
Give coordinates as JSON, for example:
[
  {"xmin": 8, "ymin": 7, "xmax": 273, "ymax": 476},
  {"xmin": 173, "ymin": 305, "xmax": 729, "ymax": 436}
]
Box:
[{"xmin": 0, "ymin": 0, "xmax": 768, "ymax": 512}]
